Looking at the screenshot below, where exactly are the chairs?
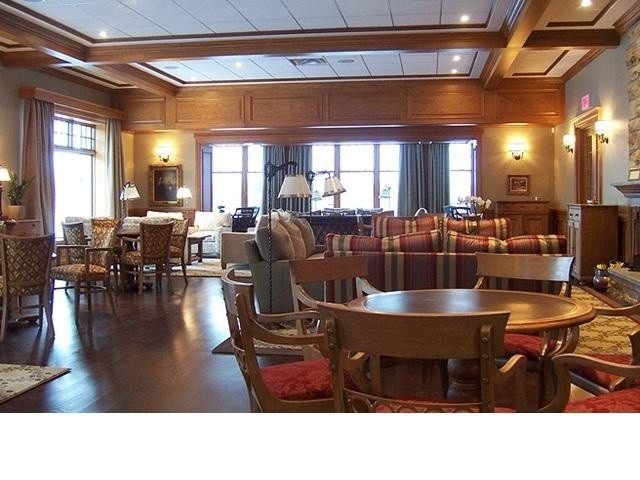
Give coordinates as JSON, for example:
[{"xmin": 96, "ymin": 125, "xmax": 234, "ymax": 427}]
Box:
[
  {"xmin": 444, "ymin": 205, "xmax": 472, "ymax": 222},
  {"xmin": 299, "ymin": 206, "xmax": 395, "ymax": 246},
  {"xmin": 559, "ymin": 298, "xmax": 639, "ymax": 397},
  {"xmin": 221, "ymin": 215, "xmax": 263, "ymax": 270},
  {"xmin": 469, "ymin": 252, "xmax": 577, "ymax": 391},
  {"xmin": 2, "ymin": 211, "xmax": 190, "ymax": 344},
  {"xmin": 283, "ymin": 255, "xmax": 389, "ymax": 380},
  {"xmin": 315, "ymin": 301, "xmax": 531, "ymax": 412},
  {"xmin": 538, "ymin": 351, "xmax": 638, "ymax": 411},
  {"xmin": 221, "ymin": 269, "xmax": 342, "ymax": 411},
  {"xmin": 186, "ymin": 211, "xmax": 233, "ymax": 259}
]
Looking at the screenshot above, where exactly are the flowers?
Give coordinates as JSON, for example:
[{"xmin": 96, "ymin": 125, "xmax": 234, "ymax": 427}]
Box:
[{"xmin": 458, "ymin": 194, "xmax": 492, "ymax": 215}]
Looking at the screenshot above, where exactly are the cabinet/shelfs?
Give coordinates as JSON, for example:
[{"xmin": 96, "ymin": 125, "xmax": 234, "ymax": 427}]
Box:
[
  {"xmin": 566, "ymin": 202, "xmax": 620, "ymax": 287},
  {"xmin": 496, "ymin": 200, "xmax": 551, "ymax": 235}
]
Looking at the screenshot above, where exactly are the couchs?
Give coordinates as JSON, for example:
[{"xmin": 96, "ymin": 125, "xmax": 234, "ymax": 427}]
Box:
[{"xmin": 244, "ymin": 214, "xmax": 330, "ymax": 315}]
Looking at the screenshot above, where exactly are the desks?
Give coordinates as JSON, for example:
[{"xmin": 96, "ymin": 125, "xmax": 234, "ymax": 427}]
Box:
[
  {"xmin": 340, "ymin": 287, "xmax": 600, "ymax": 407},
  {"xmin": 186, "ymin": 233, "xmax": 214, "ymax": 265}
]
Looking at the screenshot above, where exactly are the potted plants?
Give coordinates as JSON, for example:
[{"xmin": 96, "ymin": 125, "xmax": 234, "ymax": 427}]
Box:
[{"xmin": 6, "ymin": 169, "xmax": 39, "ymax": 221}]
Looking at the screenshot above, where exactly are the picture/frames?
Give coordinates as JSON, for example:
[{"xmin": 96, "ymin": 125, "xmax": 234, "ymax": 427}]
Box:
[
  {"xmin": 147, "ymin": 164, "xmax": 186, "ymax": 208},
  {"xmin": 508, "ymin": 174, "xmax": 530, "ymax": 196}
]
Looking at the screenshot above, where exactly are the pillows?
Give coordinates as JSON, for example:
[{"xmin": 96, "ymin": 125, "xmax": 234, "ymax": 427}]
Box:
[
  {"xmin": 255, "ymin": 209, "xmax": 316, "ymax": 263},
  {"xmin": 321, "ymin": 214, "xmax": 567, "ymax": 253}
]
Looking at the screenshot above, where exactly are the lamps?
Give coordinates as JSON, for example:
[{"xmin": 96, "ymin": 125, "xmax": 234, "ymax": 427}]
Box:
[
  {"xmin": 119, "ymin": 180, "xmax": 141, "ymax": 216},
  {"xmin": 261, "ymin": 158, "xmax": 312, "ymax": 334},
  {"xmin": 509, "ymin": 140, "xmax": 527, "ymax": 160},
  {"xmin": 593, "ymin": 120, "xmax": 611, "ymax": 144},
  {"xmin": 305, "ymin": 167, "xmax": 346, "ymax": 211},
  {"xmin": 0, "ymin": 164, "xmax": 11, "ymax": 219},
  {"xmin": 563, "ymin": 134, "xmax": 573, "ymax": 155}
]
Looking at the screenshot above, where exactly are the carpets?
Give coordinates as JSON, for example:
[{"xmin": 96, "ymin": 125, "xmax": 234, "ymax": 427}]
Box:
[
  {"xmin": 142, "ymin": 258, "xmax": 237, "ymax": 278},
  {"xmin": 0, "ymin": 363, "xmax": 72, "ymax": 407},
  {"xmin": 210, "ymin": 284, "xmax": 639, "ymax": 411}
]
